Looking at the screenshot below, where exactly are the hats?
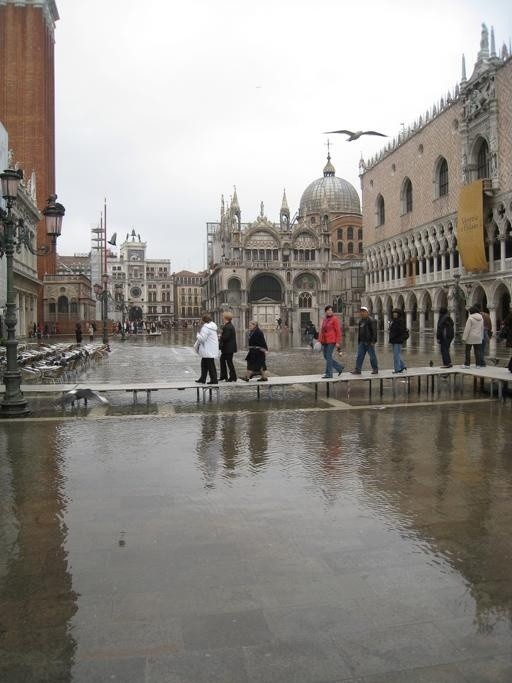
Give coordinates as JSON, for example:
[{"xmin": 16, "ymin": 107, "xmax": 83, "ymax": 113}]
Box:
[{"xmin": 359, "ymin": 306, "xmax": 369, "ymax": 313}]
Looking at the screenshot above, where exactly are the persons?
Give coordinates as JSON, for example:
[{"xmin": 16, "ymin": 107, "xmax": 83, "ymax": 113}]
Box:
[
  {"xmin": 112, "ymin": 319, "xmax": 156, "ymax": 336},
  {"xmin": 193, "ymin": 314, "xmax": 219, "ymax": 384},
  {"xmin": 320, "ymin": 411, "xmax": 453, "ymax": 473},
  {"xmin": 76, "ymin": 323, "xmax": 82, "ymax": 346},
  {"xmin": 28, "ymin": 320, "xmax": 61, "ymax": 337},
  {"xmin": 460, "ymin": 302, "xmax": 512, "ymax": 373},
  {"xmin": 238, "ymin": 320, "xmax": 268, "ymax": 382},
  {"xmin": 304, "ymin": 320, "xmax": 316, "ymax": 349},
  {"xmin": 88, "ymin": 324, "xmax": 93, "ymax": 341},
  {"xmin": 389, "ymin": 308, "xmax": 407, "ymax": 373},
  {"xmin": 217, "ymin": 312, "xmax": 237, "ymax": 382},
  {"xmin": 196, "ymin": 412, "xmax": 268, "ymax": 489},
  {"xmin": 436, "ymin": 306, "xmax": 454, "ymax": 368},
  {"xmin": 318, "ymin": 305, "xmax": 344, "ymax": 379},
  {"xmin": 350, "ymin": 306, "xmax": 378, "ymax": 374}
]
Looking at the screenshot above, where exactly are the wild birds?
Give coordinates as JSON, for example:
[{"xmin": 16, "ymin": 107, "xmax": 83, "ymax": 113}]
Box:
[
  {"xmin": 57, "ymin": 389, "xmax": 111, "ymax": 404},
  {"xmin": 324, "ymin": 128, "xmax": 389, "ymax": 142}
]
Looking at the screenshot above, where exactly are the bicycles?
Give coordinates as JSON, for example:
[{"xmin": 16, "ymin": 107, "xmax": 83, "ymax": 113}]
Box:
[{"xmin": 1, "ymin": 342, "xmax": 110, "ymax": 384}]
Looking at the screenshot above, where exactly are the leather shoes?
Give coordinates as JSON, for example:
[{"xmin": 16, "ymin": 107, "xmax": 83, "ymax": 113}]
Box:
[
  {"xmin": 322, "ymin": 375, "xmax": 333, "ymax": 379},
  {"xmin": 227, "ymin": 377, "xmax": 237, "ymax": 381},
  {"xmin": 217, "ymin": 376, "xmax": 228, "ymax": 381},
  {"xmin": 257, "ymin": 377, "xmax": 268, "ymax": 382},
  {"xmin": 195, "ymin": 379, "xmax": 206, "ymax": 384},
  {"xmin": 239, "ymin": 376, "xmax": 249, "ymax": 382},
  {"xmin": 206, "ymin": 380, "xmax": 219, "ymax": 384},
  {"xmin": 338, "ymin": 366, "xmax": 344, "ymax": 376}
]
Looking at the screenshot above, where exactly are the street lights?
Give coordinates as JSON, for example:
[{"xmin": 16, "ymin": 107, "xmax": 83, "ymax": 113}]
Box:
[
  {"xmin": 0, "ymin": 168, "xmax": 66, "ymax": 417},
  {"xmin": 95, "ymin": 272, "xmax": 124, "ymax": 351}
]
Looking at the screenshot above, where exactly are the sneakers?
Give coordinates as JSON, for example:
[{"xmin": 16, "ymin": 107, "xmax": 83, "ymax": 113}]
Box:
[
  {"xmin": 350, "ymin": 369, "xmax": 361, "ymax": 374},
  {"xmin": 440, "ymin": 363, "xmax": 486, "ymax": 368},
  {"xmin": 371, "ymin": 368, "xmax": 378, "ymax": 374}
]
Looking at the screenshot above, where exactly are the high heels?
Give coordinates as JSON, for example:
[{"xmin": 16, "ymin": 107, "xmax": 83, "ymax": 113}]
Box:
[
  {"xmin": 400, "ymin": 367, "xmax": 407, "ymax": 372},
  {"xmin": 392, "ymin": 370, "xmax": 402, "ymax": 374}
]
